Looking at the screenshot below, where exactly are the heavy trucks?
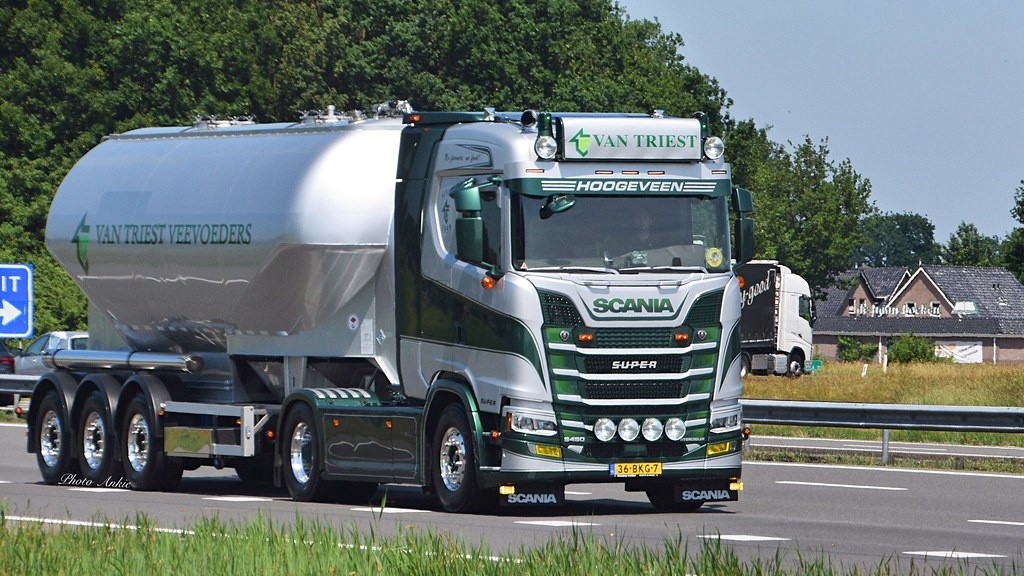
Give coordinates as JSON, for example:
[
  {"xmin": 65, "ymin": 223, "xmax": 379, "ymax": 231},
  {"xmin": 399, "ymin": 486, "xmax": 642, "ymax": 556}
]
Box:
[
  {"xmin": 730, "ymin": 258, "xmax": 817, "ymax": 377},
  {"xmin": 27, "ymin": 97, "xmax": 755, "ymax": 512}
]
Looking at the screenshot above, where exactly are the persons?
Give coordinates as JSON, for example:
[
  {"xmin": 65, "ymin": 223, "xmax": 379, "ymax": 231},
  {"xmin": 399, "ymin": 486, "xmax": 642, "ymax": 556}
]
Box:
[{"xmin": 622, "ymin": 205, "xmax": 668, "ymax": 249}]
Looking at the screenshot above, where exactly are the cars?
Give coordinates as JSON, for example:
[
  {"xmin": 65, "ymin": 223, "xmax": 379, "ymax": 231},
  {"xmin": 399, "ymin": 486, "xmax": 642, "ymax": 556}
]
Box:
[
  {"xmin": 0, "ymin": 338, "xmax": 31, "ymax": 406},
  {"xmin": 14, "ymin": 331, "xmax": 91, "ymax": 377}
]
